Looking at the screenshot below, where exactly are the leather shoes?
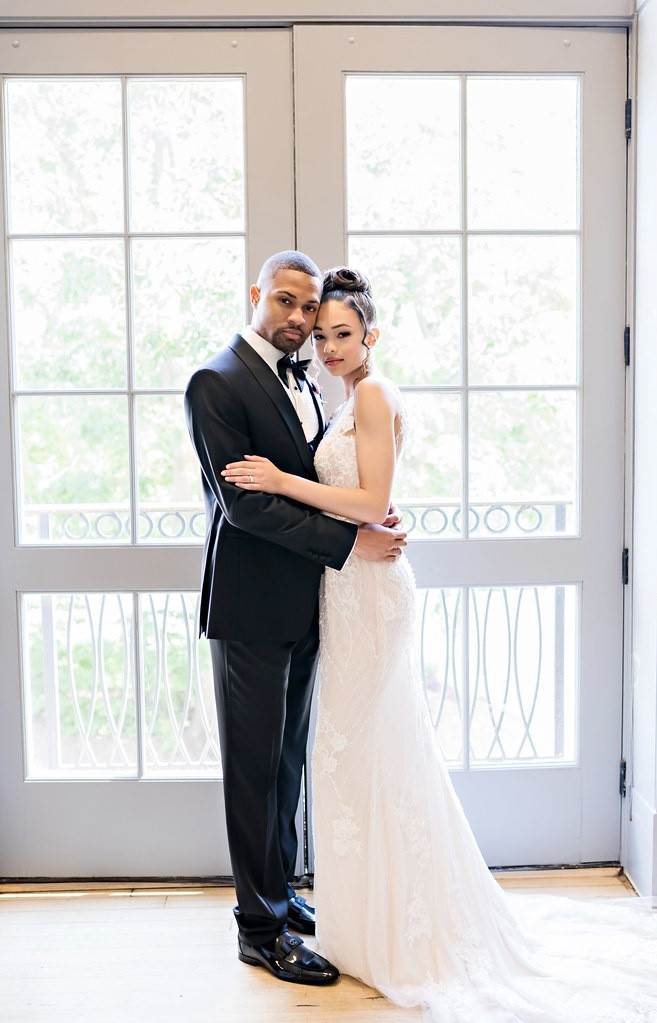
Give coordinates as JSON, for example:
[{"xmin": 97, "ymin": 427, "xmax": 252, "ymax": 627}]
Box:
[
  {"xmin": 286, "ymin": 896, "xmax": 315, "ymax": 937},
  {"xmin": 238, "ymin": 930, "xmax": 340, "ymax": 986}
]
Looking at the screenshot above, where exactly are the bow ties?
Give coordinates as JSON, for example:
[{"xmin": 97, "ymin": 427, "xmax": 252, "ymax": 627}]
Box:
[{"xmin": 277, "ymin": 352, "xmax": 312, "ymax": 393}]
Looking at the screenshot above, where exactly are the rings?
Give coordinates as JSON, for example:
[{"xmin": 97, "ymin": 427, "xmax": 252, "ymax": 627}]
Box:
[{"xmin": 250, "ymin": 476, "xmax": 253, "ymax": 482}]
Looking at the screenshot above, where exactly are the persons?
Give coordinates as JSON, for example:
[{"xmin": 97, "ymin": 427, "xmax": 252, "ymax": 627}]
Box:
[
  {"xmin": 219, "ymin": 268, "xmax": 657, "ymax": 1023},
  {"xmin": 183, "ymin": 251, "xmax": 408, "ymax": 986}
]
